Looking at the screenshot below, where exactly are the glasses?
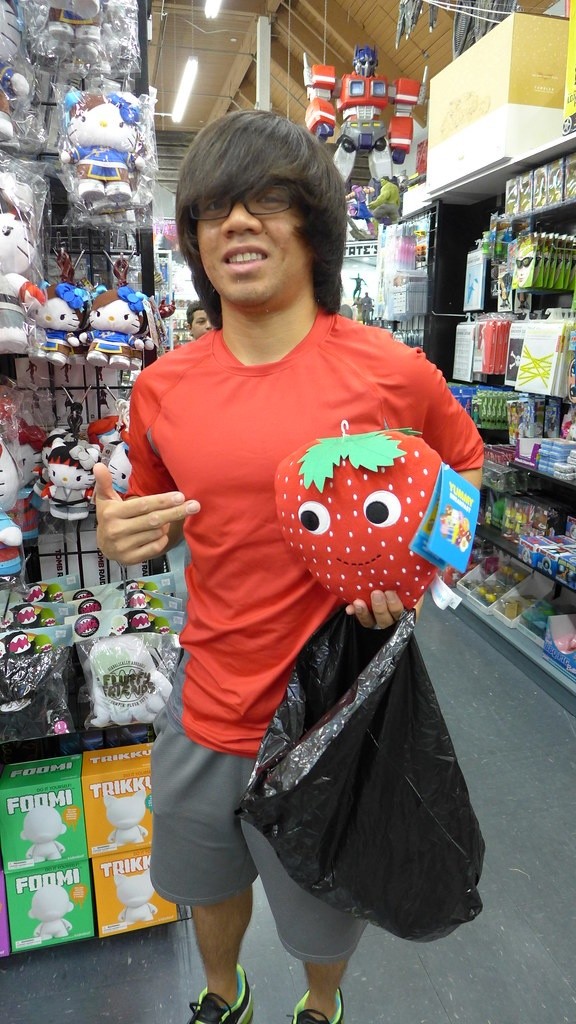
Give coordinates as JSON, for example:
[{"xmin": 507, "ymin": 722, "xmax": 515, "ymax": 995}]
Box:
[{"xmin": 189, "ymin": 184, "xmax": 295, "ymax": 220}]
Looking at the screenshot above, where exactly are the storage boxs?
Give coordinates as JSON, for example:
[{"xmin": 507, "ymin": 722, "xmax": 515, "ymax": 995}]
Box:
[
  {"xmin": 92, "ymin": 847, "xmax": 178, "ymax": 940},
  {"xmin": 4, "ymin": 857, "xmax": 94, "ymax": 955},
  {"xmin": 536, "ymin": 546, "xmax": 572, "ymax": 579},
  {"xmin": 80, "ymin": 741, "xmax": 154, "ymax": 859},
  {"xmin": 456, "ymin": 552, "xmax": 511, "ymax": 596},
  {"xmin": 492, "ymin": 570, "xmax": 555, "ymax": 630},
  {"xmin": 555, "ymin": 555, "xmax": 576, "ymax": 592},
  {"xmin": 518, "ymin": 536, "xmax": 557, "ymax": 569},
  {"xmin": 426, "ymin": 12, "xmax": 568, "ymax": 196},
  {"xmin": 565, "ymin": 515, "xmax": 576, "ymax": 541},
  {"xmin": 517, "ymin": 587, "xmax": 576, "ymax": 650},
  {"xmin": 544, "ymin": 535, "xmax": 576, "ymax": 547},
  {"xmin": 562, "ymin": 0, "xmax": 576, "ymax": 136},
  {"xmin": 542, "ymin": 613, "xmax": 576, "ymax": 685},
  {"xmin": 1, "ymin": 754, "xmax": 88, "ymax": 875},
  {"xmin": 466, "ymin": 557, "xmax": 533, "ymax": 616},
  {"xmin": 514, "ymin": 438, "xmax": 547, "ymax": 469},
  {"xmin": 560, "ymin": 544, "xmax": 576, "ymax": 556}
]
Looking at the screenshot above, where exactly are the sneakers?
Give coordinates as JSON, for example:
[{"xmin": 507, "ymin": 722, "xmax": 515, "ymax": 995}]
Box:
[
  {"xmin": 286, "ymin": 986, "xmax": 344, "ymax": 1024},
  {"xmin": 186, "ymin": 965, "xmax": 254, "ymax": 1024}
]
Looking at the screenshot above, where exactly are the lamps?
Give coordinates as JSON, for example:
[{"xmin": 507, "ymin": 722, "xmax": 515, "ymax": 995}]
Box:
[{"xmin": 171, "ymin": 1, "xmax": 199, "ymax": 124}]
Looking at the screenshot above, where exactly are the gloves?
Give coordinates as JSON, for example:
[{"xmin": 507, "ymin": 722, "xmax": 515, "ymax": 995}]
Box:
[{"xmin": 81, "ymin": 633, "xmax": 174, "ymax": 728}]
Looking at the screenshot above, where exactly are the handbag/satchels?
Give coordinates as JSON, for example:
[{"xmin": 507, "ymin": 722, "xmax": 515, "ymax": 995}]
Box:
[{"xmin": 234, "ymin": 600, "xmax": 486, "ymax": 943}]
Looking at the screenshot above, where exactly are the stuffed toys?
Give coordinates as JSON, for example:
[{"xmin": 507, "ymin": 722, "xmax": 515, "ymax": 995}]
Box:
[
  {"xmin": 0, "ymin": 1, "xmax": 175, "ymax": 748},
  {"xmin": 272, "ymin": 426, "xmax": 442, "ymax": 610}
]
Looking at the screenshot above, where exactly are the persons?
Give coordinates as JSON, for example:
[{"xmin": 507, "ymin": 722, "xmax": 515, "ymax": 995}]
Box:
[
  {"xmin": 343, "ymin": 183, "xmax": 376, "ymax": 236},
  {"xmin": 184, "ymin": 302, "xmax": 215, "ymax": 340},
  {"xmin": 174, "ymin": 333, "xmax": 183, "ymax": 349},
  {"xmin": 300, "ymin": 41, "xmax": 431, "ymax": 225},
  {"xmin": 91, "ymin": 104, "xmax": 486, "ymax": 1024},
  {"xmin": 368, "ymin": 176, "xmax": 401, "ymax": 239},
  {"xmin": 351, "ymin": 271, "xmax": 377, "ymax": 329}
]
